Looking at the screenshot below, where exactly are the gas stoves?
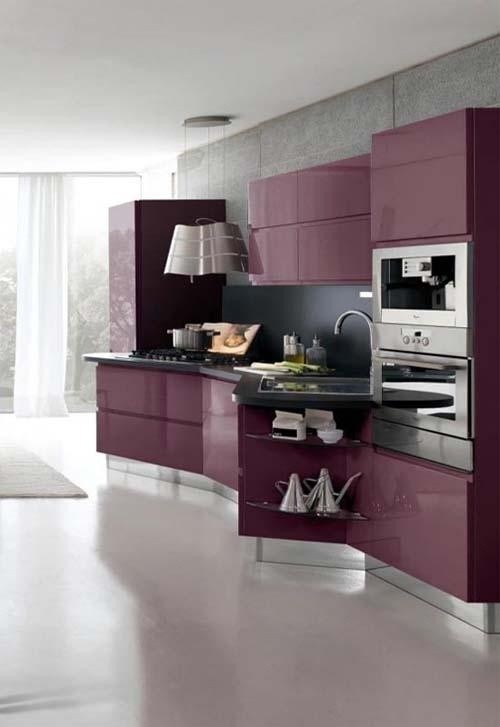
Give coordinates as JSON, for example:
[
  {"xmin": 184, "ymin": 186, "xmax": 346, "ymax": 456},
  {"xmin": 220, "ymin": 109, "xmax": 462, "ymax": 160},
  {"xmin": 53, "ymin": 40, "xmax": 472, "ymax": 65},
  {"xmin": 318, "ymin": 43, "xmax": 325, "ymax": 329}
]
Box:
[{"xmin": 128, "ymin": 348, "xmax": 257, "ymax": 366}]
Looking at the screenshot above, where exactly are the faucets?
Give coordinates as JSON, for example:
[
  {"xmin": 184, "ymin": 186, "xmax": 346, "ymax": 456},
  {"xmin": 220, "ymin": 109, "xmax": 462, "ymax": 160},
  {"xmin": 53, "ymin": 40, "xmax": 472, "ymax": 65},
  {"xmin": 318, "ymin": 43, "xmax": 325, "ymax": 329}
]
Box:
[{"xmin": 333, "ymin": 310, "xmax": 374, "ymax": 393}]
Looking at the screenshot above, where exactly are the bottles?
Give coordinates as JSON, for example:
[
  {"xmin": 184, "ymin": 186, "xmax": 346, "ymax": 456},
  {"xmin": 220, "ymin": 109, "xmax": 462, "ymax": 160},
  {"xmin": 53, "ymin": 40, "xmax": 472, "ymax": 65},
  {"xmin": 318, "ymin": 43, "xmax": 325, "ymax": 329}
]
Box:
[
  {"xmin": 305, "ymin": 331, "xmax": 327, "ymax": 367},
  {"xmin": 284, "ymin": 332, "xmax": 304, "ymax": 362}
]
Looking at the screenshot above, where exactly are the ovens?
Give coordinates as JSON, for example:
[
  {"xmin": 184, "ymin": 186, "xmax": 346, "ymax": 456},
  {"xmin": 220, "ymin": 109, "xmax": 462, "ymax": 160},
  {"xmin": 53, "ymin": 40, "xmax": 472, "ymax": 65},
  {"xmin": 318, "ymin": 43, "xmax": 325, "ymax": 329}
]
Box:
[{"xmin": 372, "ymin": 246, "xmax": 474, "ymax": 471}]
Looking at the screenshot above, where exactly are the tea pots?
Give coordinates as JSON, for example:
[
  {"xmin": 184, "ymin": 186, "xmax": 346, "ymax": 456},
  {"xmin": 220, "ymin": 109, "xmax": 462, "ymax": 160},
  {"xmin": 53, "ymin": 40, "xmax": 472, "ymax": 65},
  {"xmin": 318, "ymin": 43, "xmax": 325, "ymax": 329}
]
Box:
[
  {"xmin": 304, "ymin": 466, "xmax": 364, "ymax": 515},
  {"xmin": 275, "ymin": 473, "xmax": 326, "ymax": 516}
]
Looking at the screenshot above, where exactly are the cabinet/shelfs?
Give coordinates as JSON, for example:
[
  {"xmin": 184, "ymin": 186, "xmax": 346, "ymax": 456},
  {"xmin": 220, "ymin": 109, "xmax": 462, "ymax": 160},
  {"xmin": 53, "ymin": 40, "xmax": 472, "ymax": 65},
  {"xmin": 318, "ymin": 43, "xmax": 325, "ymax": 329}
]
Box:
[
  {"xmin": 370, "ymin": 107, "xmax": 471, "ymax": 245},
  {"xmin": 246, "ymin": 151, "xmax": 371, "ymax": 285},
  {"xmin": 361, "ymin": 432, "xmax": 425, "ymax": 521},
  {"xmin": 363, "ymin": 448, "xmax": 474, "ymax": 602},
  {"xmin": 107, "ymin": 199, "xmax": 225, "ymax": 353},
  {"xmin": 245, "ymin": 430, "xmax": 368, "ymax": 522},
  {"xmin": 92, "ymin": 363, "xmax": 238, "ymax": 505}
]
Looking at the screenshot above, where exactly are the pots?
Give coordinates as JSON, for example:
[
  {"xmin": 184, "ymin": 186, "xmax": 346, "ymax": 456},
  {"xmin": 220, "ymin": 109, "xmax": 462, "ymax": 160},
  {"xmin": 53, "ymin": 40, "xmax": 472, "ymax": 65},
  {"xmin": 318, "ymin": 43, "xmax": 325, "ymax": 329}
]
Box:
[{"xmin": 167, "ymin": 327, "xmax": 220, "ymax": 351}]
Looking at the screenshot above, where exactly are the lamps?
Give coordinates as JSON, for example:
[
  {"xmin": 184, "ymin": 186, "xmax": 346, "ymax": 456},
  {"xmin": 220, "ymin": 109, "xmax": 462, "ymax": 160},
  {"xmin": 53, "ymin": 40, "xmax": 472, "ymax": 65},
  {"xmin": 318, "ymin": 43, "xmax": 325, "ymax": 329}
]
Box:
[{"xmin": 0, "ymin": 445, "xmax": 90, "ymax": 498}]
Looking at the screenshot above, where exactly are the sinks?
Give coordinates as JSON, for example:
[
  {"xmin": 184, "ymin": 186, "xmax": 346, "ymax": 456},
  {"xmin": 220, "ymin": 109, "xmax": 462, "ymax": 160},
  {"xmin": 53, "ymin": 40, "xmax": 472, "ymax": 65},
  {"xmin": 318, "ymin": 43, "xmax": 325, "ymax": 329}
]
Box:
[{"xmin": 257, "ymin": 373, "xmax": 371, "ymax": 395}]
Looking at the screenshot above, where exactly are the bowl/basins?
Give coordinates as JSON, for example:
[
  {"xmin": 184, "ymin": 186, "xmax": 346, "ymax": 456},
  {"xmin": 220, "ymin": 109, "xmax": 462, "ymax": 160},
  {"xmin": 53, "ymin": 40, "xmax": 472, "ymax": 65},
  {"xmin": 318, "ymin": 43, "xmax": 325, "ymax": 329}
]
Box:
[{"xmin": 317, "ymin": 429, "xmax": 345, "ymax": 445}]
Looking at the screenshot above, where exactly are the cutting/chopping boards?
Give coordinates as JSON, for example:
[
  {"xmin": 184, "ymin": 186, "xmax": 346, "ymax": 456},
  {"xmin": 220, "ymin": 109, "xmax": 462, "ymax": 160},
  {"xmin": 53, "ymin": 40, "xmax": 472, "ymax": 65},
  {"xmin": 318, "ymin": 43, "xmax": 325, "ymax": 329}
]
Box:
[{"xmin": 233, "ymin": 365, "xmax": 328, "ymax": 379}]
